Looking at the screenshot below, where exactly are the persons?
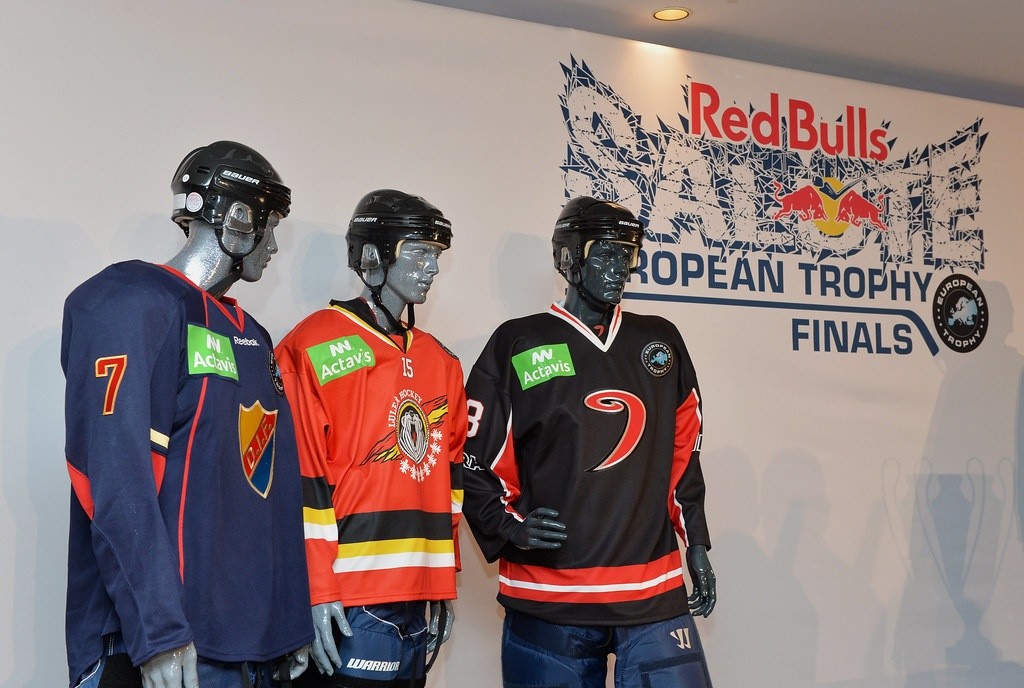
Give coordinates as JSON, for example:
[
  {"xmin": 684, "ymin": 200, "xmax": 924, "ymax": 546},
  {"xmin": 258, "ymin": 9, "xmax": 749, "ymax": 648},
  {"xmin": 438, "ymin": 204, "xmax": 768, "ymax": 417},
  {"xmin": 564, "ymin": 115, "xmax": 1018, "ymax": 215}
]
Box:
[
  {"xmin": 462, "ymin": 195, "xmax": 717, "ymax": 688},
  {"xmin": 269, "ymin": 187, "xmax": 463, "ymax": 688},
  {"xmin": 61, "ymin": 139, "xmax": 314, "ymax": 686}
]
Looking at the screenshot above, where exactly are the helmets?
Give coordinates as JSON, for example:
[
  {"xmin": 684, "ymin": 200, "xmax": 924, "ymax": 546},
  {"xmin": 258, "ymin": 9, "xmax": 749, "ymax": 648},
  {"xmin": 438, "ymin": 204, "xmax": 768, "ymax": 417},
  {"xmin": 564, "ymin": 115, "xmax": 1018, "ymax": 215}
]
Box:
[
  {"xmin": 552, "ymin": 196, "xmax": 644, "ymax": 273},
  {"xmin": 171, "ymin": 141, "xmax": 291, "ymax": 258},
  {"xmin": 345, "ymin": 189, "xmax": 452, "ymax": 268}
]
